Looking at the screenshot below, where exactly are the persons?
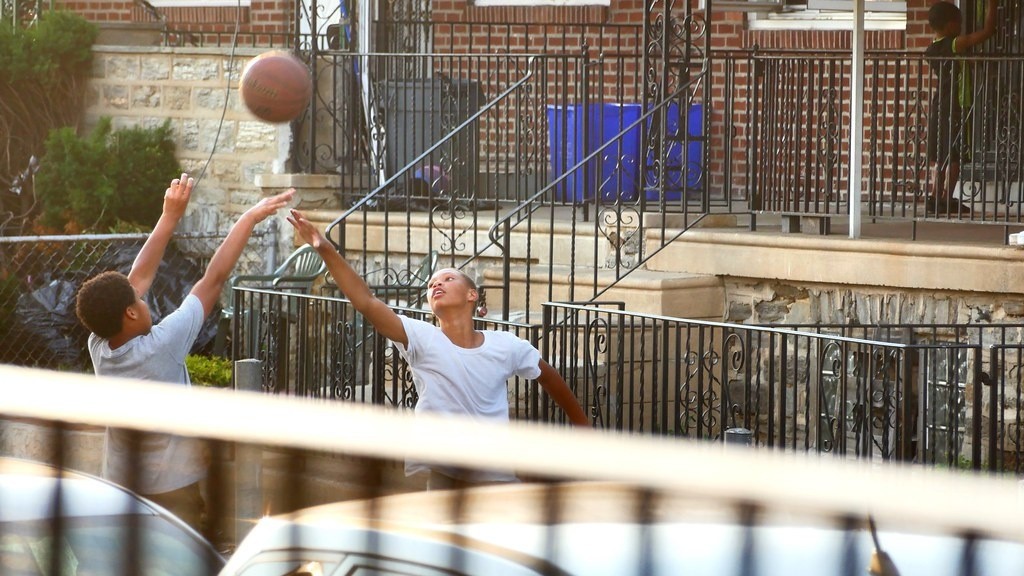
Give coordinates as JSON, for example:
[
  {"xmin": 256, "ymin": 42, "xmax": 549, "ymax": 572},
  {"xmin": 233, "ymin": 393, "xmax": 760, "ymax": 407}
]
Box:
[
  {"xmin": 286, "ymin": 209, "xmax": 590, "ymax": 492},
  {"xmin": 76, "ymin": 172, "xmax": 296, "ymax": 529},
  {"xmin": 924, "ymin": 0, "xmax": 998, "ymax": 212}
]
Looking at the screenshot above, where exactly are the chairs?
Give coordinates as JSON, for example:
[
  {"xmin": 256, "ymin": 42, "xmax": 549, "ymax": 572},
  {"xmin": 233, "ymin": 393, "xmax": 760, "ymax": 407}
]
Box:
[{"xmin": 211, "ymin": 243, "xmax": 328, "ymax": 389}]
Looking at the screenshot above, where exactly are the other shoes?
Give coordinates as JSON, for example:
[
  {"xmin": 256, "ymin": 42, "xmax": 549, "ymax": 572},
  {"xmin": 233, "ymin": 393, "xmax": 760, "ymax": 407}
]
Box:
[
  {"xmin": 938, "ymin": 197, "xmax": 970, "ymax": 212},
  {"xmin": 927, "ymin": 196, "xmax": 936, "ymax": 213}
]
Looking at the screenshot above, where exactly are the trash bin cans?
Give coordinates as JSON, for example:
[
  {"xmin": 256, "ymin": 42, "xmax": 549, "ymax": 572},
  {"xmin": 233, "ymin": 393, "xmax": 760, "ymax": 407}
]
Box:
[
  {"xmin": 548, "ymin": 103, "xmax": 643, "ymax": 203},
  {"xmin": 386, "ymin": 77, "xmax": 481, "ymax": 211},
  {"xmin": 639, "ymin": 103, "xmax": 705, "ymax": 200}
]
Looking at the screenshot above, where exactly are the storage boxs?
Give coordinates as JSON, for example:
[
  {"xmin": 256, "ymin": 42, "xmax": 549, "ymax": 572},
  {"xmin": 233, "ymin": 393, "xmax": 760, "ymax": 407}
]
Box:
[{"xmin": 546, "ymin": 102, "xmax": 702, "ymax": 200}]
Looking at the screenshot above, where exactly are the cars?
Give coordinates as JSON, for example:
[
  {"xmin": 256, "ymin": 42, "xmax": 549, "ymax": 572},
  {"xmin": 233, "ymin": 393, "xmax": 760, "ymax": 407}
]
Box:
[
  {"xmin": 0, "ymin": 454, "xmax": 229, "ymax": 576},
  {"xmin": 214, "ymin": 481, "xmax": 1024, "ymax": 576}
]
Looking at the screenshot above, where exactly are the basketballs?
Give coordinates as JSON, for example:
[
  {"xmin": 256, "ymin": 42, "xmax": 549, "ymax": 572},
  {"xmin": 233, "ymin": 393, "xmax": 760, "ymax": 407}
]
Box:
[{"xmin": 242, "ymin": 51, "xmax": 313, "ymax": 125}]
